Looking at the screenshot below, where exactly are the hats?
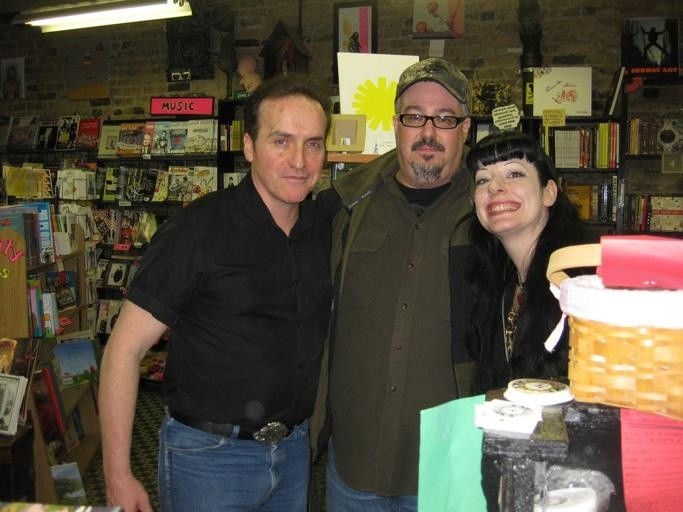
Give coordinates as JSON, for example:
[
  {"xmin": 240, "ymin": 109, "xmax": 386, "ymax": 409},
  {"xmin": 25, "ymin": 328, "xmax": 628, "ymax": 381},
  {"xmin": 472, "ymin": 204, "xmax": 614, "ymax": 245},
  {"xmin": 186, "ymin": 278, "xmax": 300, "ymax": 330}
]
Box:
[{"xmin": 394, "ymin": 57, "xmax": 469, "ymax": 103}]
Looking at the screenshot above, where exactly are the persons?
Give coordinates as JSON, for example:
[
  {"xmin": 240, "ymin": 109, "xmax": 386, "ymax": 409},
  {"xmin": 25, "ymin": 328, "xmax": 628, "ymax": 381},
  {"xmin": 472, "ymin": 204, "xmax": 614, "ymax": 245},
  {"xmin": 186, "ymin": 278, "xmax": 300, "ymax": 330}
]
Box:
[
  {"xmin": 348, "ymin": 32, "xmax": 362, "ymax": 52},
  {"xmin": 307, "ymin": 56, "xmax": 478, "ymax": 512},
  {"xmin": 465, "ymin": 130, "xmax": 601, "ymax": 395},
  {"xmin": 95, "ymin": 73, "xmax": 334, "ymax": 512}
]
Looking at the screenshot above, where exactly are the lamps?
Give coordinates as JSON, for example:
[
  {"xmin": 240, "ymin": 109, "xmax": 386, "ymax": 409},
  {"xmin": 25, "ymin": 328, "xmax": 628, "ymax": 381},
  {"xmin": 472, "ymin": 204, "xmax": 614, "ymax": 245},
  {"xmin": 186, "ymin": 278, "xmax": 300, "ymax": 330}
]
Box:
[{"xmin": 12, "ymin": 0, "xmax": 193, "ymax": 32}]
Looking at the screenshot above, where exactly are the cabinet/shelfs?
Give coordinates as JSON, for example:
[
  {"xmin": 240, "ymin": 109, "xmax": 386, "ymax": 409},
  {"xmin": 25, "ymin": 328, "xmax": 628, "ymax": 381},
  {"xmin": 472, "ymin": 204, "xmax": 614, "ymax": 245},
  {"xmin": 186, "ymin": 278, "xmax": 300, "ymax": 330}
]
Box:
[
  {"xmin": 620, "ymin": 69, "xmax": 683, "ymax": 237},
  {"xmin": 310, "ymin": 155, "xmax": 381, "ymax": 201},
  {"xmin": 218, "ymin": 100, "xmax": 248, "ymax": 190},
  {"xmin": 1, "ymin": 224, "xmax": 98, "ymax": 478},
  {"xmin": 469, "ymin": 116, "xmax": 620, "ymax": 237},
  {"xmin": 0, "ymin": 115, "xmax": 218, "ymax": 385},
  {"xmin": 0, "ymin": 426, "xmax": 36, "ymax": 503}
]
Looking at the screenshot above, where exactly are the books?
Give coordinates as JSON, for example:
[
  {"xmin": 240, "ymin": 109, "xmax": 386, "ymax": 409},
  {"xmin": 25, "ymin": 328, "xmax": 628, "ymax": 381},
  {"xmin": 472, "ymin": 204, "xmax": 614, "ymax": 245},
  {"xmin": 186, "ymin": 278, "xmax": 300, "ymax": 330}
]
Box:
[
  {"xmin": 1, "ymin": 113, "xmax": 247, "ymax": 512},
  {"xmin": 530, "ymin": 65, "xmax": 683, "ymax": 236}
]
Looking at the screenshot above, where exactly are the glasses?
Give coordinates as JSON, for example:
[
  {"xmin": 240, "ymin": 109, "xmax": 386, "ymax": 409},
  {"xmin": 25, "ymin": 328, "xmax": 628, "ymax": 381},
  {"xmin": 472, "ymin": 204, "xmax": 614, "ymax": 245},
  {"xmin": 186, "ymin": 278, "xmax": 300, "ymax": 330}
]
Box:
[{"xmin": 398, "ymin": 114, "xmax": 463, "ymax": 129}]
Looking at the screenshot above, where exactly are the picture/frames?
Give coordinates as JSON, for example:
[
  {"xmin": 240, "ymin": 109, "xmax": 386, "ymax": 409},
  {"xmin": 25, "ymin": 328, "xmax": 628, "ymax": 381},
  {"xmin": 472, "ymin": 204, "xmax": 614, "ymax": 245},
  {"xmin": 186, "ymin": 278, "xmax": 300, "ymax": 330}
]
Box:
[
  {"xmin": 1, "ymin": 57, "xmax": 24, "ymax": 100},
  {"xmin": 623, "ymin": 17, "xmax": 680, "ymax": 68},
  {"xmin": 333, "ymin": 0, "xmax": 377, "ymax": 83},
  {"xmin": 0, "ymin": 374, "xmax": 28, "ymax": 436}
]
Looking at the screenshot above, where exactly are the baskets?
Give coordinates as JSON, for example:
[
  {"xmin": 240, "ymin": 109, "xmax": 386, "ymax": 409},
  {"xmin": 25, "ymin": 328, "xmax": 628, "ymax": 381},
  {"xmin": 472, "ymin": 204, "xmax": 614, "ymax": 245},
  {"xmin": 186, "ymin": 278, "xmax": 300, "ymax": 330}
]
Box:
[{"xmin": 546, "ymin": 233, "xmax": 682, "ymax": 422}]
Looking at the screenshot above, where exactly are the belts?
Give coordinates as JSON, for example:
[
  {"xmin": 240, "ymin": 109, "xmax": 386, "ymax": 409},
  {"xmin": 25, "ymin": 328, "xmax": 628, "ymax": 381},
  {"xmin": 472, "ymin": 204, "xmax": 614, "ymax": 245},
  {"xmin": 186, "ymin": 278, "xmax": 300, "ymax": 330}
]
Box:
[{"xmin": 182, "ymin": 415, "xmax": 296, "ymax": 443}]
